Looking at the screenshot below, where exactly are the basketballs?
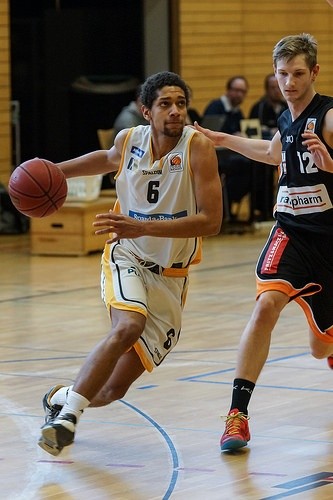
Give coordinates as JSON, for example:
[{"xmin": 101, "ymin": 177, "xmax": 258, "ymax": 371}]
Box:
[{"xmin": 8, "ymin": 157, "xmax": 68, "ymax": 220}]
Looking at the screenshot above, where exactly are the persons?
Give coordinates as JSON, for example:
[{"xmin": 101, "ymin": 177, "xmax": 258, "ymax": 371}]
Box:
[
  {"xmin": 246, "ymin": 74, "xmax": 289, "ymax": 205},
  {"xmin": 33, "ymin": 69, "xmax": 223, "ymax": 456},
  {"xmin": 111, "ymin": 79, "xmax": 150, "ymax": 190},
  {"xmin": 184, "ymin": 32, "xmax": 333, "ymax": 450},
  {"xmin": 185, "ymin": 87, "xmax": 202, "ymax": 126},
  {"xmin": 202, "ymin": 76, "xmax": 276, "ymax": 228}
]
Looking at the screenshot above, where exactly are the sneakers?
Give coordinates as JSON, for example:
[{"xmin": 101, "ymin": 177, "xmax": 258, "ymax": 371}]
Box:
[
  {"xmin": 37, "ymin": 411, "xmax": 76, "ymax": 457},
  {"xmin": 42, "ymin": 384, "xmax": 66, "ymax": 424},
  {"xmin": 220, "ymin": 408, "xmax": 251, "ymax": 450},
  {"xmin": 327, "ymin": 357, "xmax": 333, "ymax": 368}
]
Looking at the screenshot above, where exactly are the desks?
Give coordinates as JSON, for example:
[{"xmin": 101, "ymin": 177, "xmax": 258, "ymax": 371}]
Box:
[{"xmin": 31, "ymin": 190, "xmax": 119, "ymax": 257}]
